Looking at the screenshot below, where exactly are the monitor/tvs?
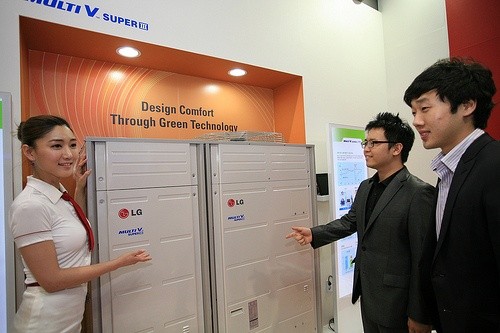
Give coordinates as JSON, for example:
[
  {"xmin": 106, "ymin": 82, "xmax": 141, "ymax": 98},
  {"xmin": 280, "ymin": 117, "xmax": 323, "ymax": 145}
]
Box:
[{"xmin": 316, "ymin": 173, "xmax": 329, "ymax": 196}]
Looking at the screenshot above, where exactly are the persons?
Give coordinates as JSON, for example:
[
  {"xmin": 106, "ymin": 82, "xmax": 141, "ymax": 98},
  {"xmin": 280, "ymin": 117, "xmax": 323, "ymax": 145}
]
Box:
[
  {"xmin": 8, "ymin": 115, "xmax": 152, "ymax": 333},
  {"xmin": 403, "ymin": 58, "xmax": 500, "ymax": 333},
  {"xmin": 286, "ymin": 110, "xmax": 436, "ymax": 333}
]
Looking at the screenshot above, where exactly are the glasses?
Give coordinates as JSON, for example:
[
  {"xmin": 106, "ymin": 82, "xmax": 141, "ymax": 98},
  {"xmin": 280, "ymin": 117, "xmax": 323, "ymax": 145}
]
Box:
[{"xmin": 361, "ymin": 140, "xmax": 399, "ymax": 149}]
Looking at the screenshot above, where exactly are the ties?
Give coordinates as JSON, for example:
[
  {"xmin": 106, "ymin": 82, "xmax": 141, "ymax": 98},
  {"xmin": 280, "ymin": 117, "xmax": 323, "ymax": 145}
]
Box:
[{"xmin": 61, "ymin": 191, "xmax": 95, "ymax": 255}]
